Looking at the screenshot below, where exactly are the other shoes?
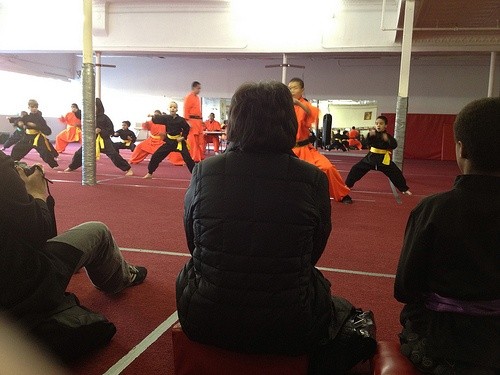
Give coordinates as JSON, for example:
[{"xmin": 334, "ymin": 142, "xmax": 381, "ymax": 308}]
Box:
[{"xmin": 215, "ymin": 152, "xmax": 219, "ymax": 155}]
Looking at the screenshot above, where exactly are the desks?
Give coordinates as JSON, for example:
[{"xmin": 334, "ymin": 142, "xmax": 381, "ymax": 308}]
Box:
[{"xmin": 204, "ymin": 131, "xmax": 226, "ymax": 154}]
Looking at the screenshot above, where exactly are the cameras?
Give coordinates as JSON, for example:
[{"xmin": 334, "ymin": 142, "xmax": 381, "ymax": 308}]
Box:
[{"xmin": 13, "ymin": 161, "xmax": 43, "ymax": 177}]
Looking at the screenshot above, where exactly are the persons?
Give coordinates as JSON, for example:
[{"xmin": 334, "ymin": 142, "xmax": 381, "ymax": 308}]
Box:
[
  {"xmin": 288, "ymin": 77, "xmax": 352, "ymax": 204},
  {"xmin": 0, "ymin": 80, "xmax": 229, "ymax": 375},
  {"xmin": 345, "ymin": 116, "xmax": 411, "ymax": 196},
  {"xmin": 317, "ymin": 126, "xmax": 371, "ymax": 152},
  {"xmin": 175, "ymin": 80, "xmax": 357, "ymax": 350},
  {"xmin": 393, "ymin": 97, "xmax": 500, "ymax": 375}
]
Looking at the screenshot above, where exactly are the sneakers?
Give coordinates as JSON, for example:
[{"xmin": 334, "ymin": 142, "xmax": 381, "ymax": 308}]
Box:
[{"xmin": 125, "ymin": 265, "xmax": 147, "ymax": 285}]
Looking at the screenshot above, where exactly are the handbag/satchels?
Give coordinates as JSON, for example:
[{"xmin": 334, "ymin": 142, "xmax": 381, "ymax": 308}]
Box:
[
  {"xmin": 25, "ymin": 291, "xmax": 116, "ymax": 361},
  {"xmin": 309, "ymin": 308, "xmax": 376, "ymax": 375}
]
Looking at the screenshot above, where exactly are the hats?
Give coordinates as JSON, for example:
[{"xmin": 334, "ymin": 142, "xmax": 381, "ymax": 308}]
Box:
[{"xmin": 122, "ymin": 121, "xmax": 131, "ymax": 127}]
